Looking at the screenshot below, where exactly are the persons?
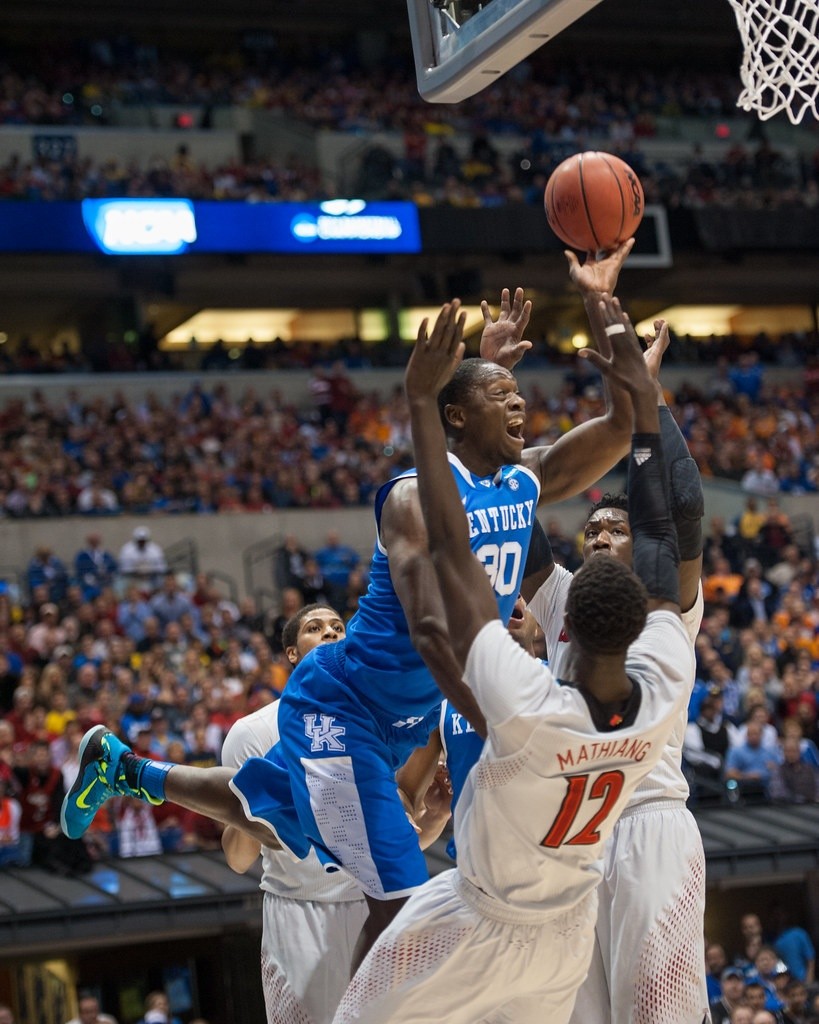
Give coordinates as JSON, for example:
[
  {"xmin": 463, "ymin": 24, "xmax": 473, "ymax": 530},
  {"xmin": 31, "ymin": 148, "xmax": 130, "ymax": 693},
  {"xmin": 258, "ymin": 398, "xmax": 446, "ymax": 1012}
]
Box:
[{"xmin": 0, "ymin": 0, "xmax": 819, "ymax": 1024}]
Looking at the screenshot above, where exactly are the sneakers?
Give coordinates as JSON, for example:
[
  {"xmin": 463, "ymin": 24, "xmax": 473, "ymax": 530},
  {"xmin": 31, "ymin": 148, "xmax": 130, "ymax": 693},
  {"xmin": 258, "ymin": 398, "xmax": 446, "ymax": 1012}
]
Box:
[{"xmin": 61, "ymin": 724, "xmax": 132, "ymax": 839}]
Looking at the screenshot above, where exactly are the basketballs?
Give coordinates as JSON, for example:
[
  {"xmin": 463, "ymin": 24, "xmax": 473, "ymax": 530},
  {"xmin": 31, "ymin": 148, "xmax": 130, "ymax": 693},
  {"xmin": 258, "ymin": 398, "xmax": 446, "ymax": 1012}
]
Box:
[{"xmin": 544, "ymin": 150, "xmax": 646, "ymax": 254}]
